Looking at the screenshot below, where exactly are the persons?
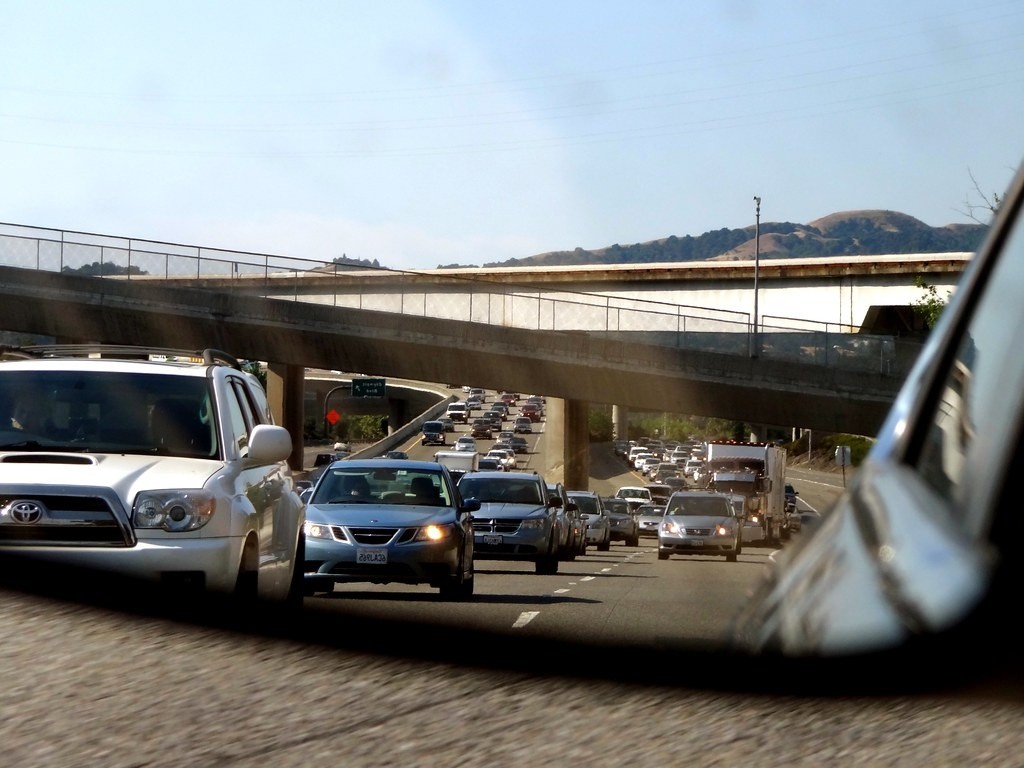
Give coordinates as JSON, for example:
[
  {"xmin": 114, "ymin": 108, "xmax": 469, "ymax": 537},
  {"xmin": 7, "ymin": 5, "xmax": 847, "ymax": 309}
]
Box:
[
  {"xmin": 350, "ymin": 481, "xmax": 364, "ymax": 496},
  {"xmin": 11, "ymin": 398, "xmax": 42, "ymax": 435},
  {"xmin": 669, "ymin": 502, "xmax": 687, "ymax": 515}
]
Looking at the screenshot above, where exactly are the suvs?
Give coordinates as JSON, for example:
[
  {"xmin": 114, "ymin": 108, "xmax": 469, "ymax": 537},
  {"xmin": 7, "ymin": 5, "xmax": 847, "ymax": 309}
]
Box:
[
  {"xmin": 447, "ymin": 470, "xmax": 563, "ymax": 576},
  {"xmin": 567, "ymin": 490, "xmax": 613, "ymax": 552},
  {"xmin": 499, "ymin": 482, "xmax": 579, "ymax": 559},
  {"xmin": 0, "ymin": 344, "xmax": 309, "ymax": 606}
]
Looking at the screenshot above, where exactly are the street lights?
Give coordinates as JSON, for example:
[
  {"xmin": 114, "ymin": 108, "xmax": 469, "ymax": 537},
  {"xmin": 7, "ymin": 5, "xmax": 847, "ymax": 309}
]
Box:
[{"xmin": 753, "ymin": 194, "xmax": 762, "ymax": 340}]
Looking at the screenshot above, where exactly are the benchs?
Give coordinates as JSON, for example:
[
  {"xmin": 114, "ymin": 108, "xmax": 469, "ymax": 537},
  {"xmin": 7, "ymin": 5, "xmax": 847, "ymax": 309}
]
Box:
[{"xmin": 370, "ymin": 491, "xmax": 415, "ymax": 504}]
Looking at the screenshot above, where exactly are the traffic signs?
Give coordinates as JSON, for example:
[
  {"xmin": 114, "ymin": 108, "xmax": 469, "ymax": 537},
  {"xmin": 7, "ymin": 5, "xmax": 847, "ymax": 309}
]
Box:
[{"xmin": 351, "ymin": 378, "xmax": 386, "ymax": 398}]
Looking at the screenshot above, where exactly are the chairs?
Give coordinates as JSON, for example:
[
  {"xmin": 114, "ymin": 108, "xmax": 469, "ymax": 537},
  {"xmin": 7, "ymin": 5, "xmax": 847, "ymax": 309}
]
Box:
[{"xmin": 410, "ymin": 477, "xmax": 432, "ymax": 495}]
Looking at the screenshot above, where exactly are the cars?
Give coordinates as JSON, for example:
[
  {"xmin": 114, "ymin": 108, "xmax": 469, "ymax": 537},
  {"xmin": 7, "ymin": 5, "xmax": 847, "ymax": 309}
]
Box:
[
  {"xmin": 615, "ymin": 437, "xmax": 707, "ymax": 498},
  {"xmin": 319, "ymin": 383, "xmax": 544, "ymax": 474},
  {"xmin": 655, "ymin": 488, "xmax": 741, "ymax": 564},
  {"xmin": 631, "ymin": 504, "xmax": 670, "ymax": 539},
  {"xmin": 612, "ymin": 486, "xmax": 652, "ymax": 507},
  {"xmin": 297, "ymin": 458, "xmax": 478, "ymax": 603},
  {"xmin": 567, "ymin": 497, "xmax": 591, "ymax": 555},
  {"xmin": 602, "ymin": 495, "xmax": 642, "ymax": 547},
  {"xmin": 783, "ymin": 481, "xmax": 805, "ymax": 538}
]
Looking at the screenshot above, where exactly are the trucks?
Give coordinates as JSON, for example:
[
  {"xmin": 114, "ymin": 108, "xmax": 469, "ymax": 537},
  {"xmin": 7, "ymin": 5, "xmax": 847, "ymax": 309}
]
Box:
[{"xmin": 705, "ymin": 439, "xmax": 787, "ymax": 545}]
